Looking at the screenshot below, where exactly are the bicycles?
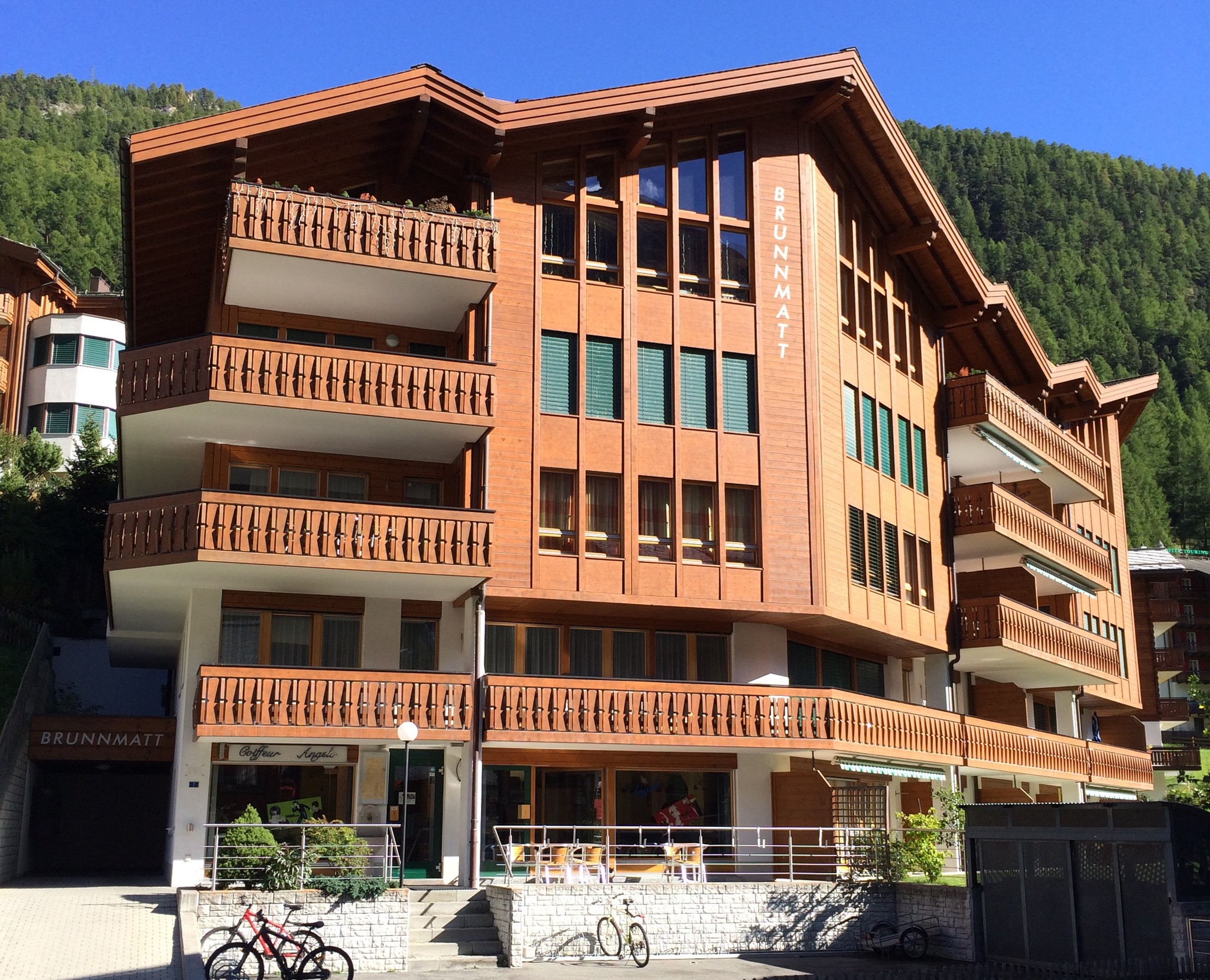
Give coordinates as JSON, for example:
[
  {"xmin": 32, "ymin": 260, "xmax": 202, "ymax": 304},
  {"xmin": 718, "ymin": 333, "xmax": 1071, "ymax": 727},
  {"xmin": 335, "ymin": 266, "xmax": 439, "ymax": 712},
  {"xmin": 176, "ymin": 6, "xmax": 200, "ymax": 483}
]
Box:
[
  {"xmin": 592, "ymin": 893, "xmax": 649, "ymax": 967},
  {"xmin": 200, "ymin": 894, "xmax": 325, "ymax": 979},
  {"xmin": 204, "ymin": 909, "xmax": 354, "ymax": 980}
]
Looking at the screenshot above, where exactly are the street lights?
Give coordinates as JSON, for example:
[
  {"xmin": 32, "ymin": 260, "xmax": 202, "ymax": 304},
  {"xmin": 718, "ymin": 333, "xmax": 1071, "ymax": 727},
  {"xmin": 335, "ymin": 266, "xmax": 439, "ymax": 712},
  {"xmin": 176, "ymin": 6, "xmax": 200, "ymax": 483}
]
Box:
[{"xmin": 398, "ymin": 721, "xmax": 418, "ymax": 887}]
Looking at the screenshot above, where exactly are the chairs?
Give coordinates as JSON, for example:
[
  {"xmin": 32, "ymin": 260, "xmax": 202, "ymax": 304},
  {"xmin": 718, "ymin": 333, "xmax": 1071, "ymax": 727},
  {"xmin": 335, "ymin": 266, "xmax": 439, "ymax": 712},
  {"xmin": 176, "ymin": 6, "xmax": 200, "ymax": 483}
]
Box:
[{"xmin": 499, "ymin": 838, "xmax": 709, "ymax": 887}]
